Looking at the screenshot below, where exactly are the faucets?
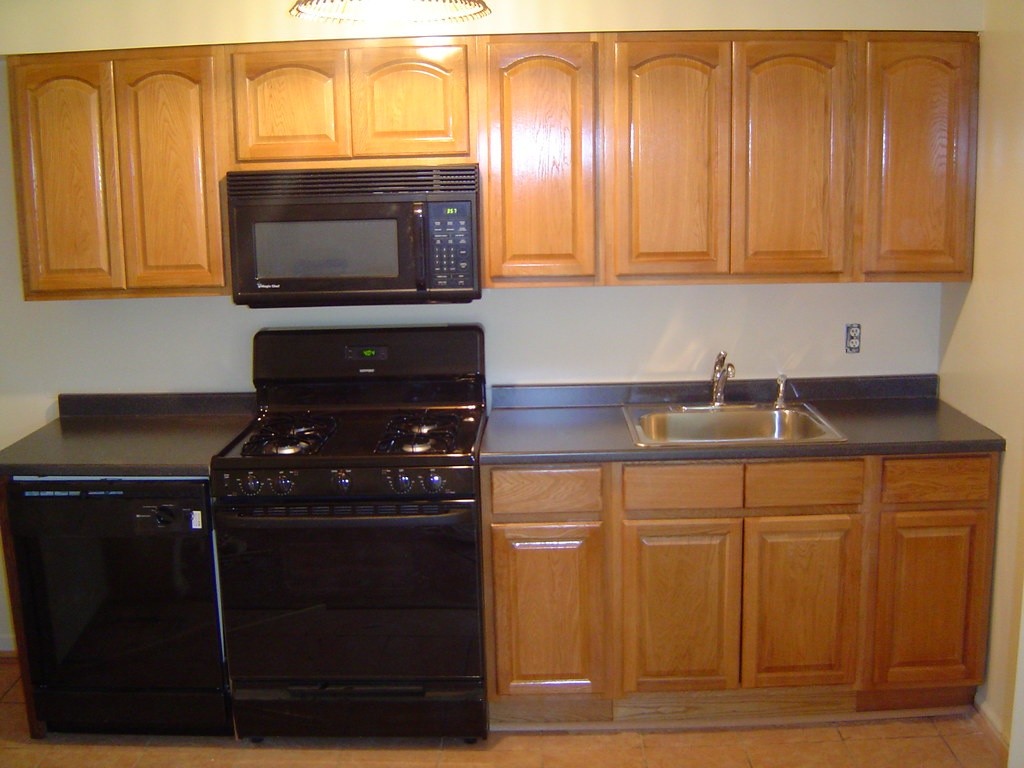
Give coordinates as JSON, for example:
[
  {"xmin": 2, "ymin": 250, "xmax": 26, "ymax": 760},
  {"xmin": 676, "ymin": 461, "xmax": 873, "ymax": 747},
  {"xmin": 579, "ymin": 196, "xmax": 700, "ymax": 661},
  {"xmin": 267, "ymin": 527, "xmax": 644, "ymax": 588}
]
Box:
[{"xmin": 712, "ymin": 350, "xmax": 735, "ymax": 407}]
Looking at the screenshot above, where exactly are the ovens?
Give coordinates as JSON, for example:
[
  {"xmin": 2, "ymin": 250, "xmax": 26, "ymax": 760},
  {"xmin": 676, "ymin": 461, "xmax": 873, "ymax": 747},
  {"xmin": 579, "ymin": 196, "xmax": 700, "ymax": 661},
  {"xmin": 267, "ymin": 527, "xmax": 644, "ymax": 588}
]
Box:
[
  {"xmin": 8, "ymin": 474, "xmax": 231, "ymax": 737},
  {"xmin": 214, "ymin": 502, "xmax": 488, "ymax": 744}
]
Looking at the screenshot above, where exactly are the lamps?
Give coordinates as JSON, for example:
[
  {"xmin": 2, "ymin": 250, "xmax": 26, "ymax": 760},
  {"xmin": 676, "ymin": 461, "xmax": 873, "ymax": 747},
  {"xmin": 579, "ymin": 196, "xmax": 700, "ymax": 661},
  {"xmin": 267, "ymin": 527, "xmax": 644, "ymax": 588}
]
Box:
[{"xmin": 289, "ymin": 0, "xmax": 492, "ymax": 32}]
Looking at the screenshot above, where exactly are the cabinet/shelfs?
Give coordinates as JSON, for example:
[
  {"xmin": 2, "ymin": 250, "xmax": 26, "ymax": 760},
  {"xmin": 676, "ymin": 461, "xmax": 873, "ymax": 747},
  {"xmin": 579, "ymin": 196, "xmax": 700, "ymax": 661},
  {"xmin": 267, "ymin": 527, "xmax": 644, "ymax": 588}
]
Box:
[
  {"xmin": 611, "ymin": 31, "xmax": 847, "ymax": 287},
  {"xmin": 226, "ymin": 35, "xmax": 478, "ymax": 169},
  {"xmin": 614, "ymin": 460, "xmax": 866, "ymax": 732},
  {"xmin": 866, "ymin": 456, "xmax": 1000, "ymax": 725},
  {"xmin": 480, "ymin": 464, "xmax": 613, "ymax": 731},
  {"xmin": 483, "ymin": 32, "xmax": 606, "ymax": 286},
  {"xmin": 849, "ymin": 31, "xmax": 979, "ymax": 282},
  {"xmin": 7, "ymin": 44, "xmax": 225, "ymax": 300}
]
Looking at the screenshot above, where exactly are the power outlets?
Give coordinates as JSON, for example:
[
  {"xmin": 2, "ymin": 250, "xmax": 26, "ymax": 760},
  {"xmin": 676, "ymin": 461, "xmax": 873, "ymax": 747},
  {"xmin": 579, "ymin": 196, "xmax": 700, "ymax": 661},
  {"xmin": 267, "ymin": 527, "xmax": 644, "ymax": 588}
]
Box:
[{"xmin": 845, "ymin": 324, "xmax": 861, "ymax": 353}]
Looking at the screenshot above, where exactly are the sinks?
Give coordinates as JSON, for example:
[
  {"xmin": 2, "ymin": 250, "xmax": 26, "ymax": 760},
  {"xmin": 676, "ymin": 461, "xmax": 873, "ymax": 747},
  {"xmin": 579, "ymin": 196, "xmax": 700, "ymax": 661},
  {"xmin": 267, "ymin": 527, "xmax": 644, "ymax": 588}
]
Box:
[{"xmin": 621, "ymin": 401, "xmax": 849, "ymax": 449}]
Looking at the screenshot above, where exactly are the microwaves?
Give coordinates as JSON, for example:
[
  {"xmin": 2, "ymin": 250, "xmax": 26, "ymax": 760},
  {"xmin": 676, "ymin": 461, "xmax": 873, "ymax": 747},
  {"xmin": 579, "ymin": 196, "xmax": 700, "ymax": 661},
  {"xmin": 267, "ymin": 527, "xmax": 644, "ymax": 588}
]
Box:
[{"xmin": 226, "ymin": 162, "xmax": 482, "ymax": 309}]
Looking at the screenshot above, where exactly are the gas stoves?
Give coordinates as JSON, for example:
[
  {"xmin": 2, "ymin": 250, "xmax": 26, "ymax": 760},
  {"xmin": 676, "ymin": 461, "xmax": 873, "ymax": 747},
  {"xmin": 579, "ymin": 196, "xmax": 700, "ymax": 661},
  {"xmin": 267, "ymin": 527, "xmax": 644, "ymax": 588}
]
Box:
[{"xmin": 210, "ymin": 322, "xmax": 487, "ymax": 502}]
